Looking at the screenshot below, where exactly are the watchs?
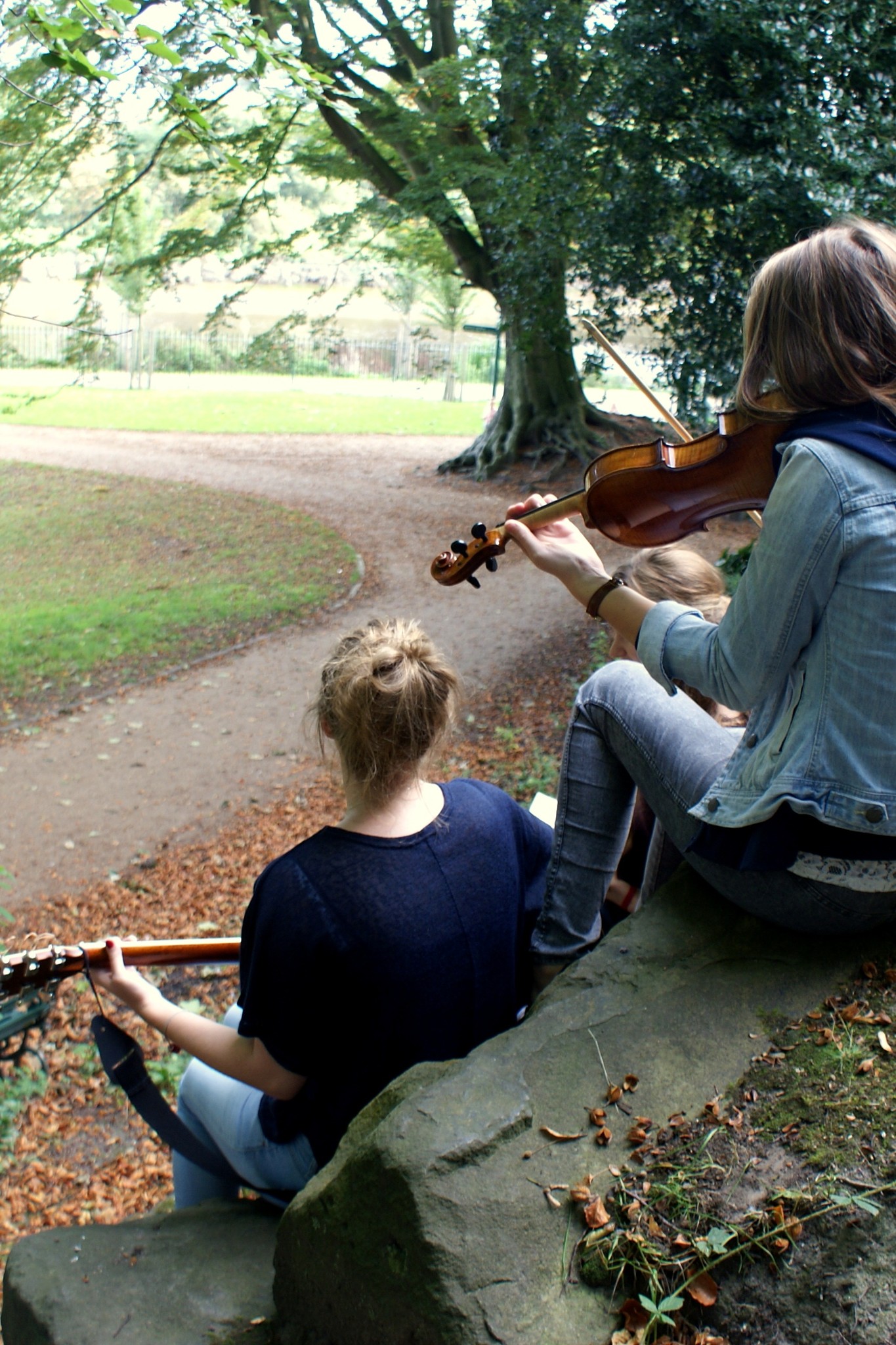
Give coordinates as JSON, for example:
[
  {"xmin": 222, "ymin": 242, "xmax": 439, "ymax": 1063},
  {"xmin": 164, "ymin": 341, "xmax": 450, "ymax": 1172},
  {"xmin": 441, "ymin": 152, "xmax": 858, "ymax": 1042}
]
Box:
[{"xmin": 585, "ymin": 577, "xmax": 627, "ymax": 622}]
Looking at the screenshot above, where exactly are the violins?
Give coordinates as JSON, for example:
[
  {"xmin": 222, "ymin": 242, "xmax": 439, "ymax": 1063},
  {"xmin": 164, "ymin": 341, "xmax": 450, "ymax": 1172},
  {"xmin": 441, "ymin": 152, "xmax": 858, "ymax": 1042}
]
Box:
[{"xmin": 432, "ymin": 386, "xmax": 811, "ymax": 588}]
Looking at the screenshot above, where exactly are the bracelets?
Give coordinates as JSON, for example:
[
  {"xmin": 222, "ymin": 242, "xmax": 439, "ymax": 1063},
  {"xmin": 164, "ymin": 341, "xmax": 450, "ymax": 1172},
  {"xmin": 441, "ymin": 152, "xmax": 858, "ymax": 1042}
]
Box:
[{"xmin": 620, "ymin": 885, "xmax": 637, "ymax": 911}]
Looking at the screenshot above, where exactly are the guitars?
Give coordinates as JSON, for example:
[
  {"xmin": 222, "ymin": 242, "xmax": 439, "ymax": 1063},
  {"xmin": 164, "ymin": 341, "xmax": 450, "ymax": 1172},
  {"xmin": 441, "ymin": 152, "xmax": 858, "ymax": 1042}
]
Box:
[{"xmin": 0, "ymin": 938, "xmax": 243, "ymax": 990}]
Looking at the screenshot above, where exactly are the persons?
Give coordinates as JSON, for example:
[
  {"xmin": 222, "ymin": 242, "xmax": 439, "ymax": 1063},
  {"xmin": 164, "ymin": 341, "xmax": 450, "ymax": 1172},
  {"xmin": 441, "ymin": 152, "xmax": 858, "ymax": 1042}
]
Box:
[
  {"xmin": 600, "ymin": 541, "xmax": 750, "ymax": 942},
  {"xmin": 504, "ymin": 212, "xmax": 896, "ymax": 972},
  {"xmin": 82, "ymin": 614, "xmax": 556, "ymax": 1210}
]
